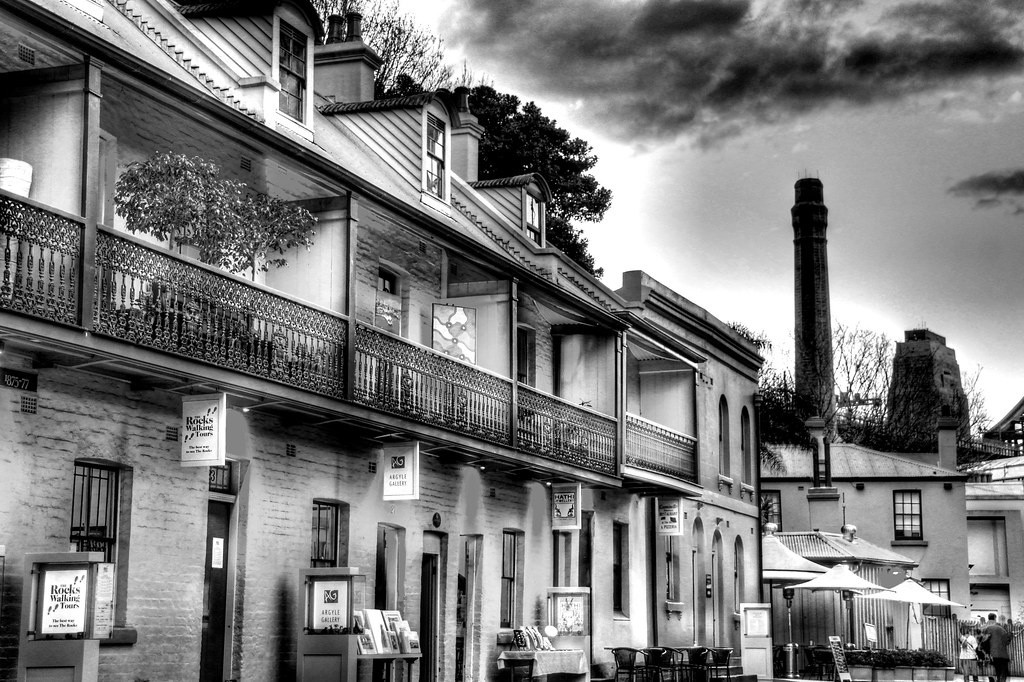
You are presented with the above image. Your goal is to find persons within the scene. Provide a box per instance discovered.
[981,622,1012,682]
[958,625,979,682]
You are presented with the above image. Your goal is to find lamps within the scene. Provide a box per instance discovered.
[969,565,974,569]
[856,483,865,490]
[944,483,952,490]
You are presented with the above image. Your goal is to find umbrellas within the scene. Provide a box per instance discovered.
[855,578,964,650]
[784,564,897,642]
[761,535,832,582]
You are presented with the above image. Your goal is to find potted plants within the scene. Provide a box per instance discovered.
[844,646,956,682]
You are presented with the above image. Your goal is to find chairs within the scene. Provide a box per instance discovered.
[773,643,837,681]
[611,647,734,682]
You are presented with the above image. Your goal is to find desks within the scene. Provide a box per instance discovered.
[497,650,590,682]
[356,652,423,682]
[648,646,702,682]
[816,649,867,682]
